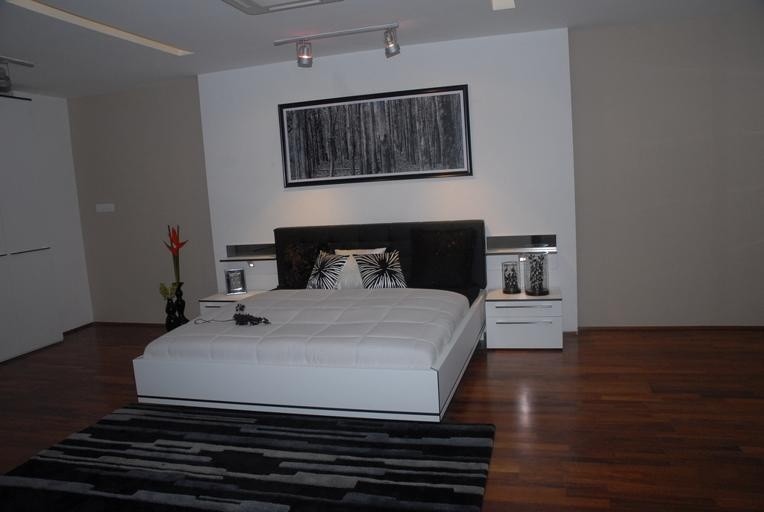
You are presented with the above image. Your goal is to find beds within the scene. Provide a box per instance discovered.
[132,220,488,425]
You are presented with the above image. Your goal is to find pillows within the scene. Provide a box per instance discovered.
[306,248,408,290]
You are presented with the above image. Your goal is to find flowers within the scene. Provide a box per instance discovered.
[162,224,188,284]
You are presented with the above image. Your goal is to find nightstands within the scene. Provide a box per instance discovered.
[197,290,267,318]
[484,289,564,350]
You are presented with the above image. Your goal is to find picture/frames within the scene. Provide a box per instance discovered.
[224,268,247,295]
[277,83,473,188]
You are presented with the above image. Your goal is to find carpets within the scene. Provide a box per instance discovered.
[1,400,498,511]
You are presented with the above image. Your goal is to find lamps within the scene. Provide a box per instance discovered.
[382,25,399,49]
[295,36,313,59]
